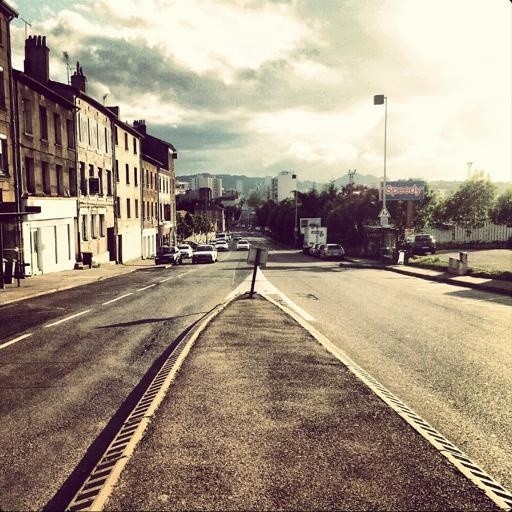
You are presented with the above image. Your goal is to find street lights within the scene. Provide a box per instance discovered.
[374,95,387,208]
[293,175,299,249]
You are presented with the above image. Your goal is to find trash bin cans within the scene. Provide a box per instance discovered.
[83,253,93,268]
[397,250,405,265]
[15,262,25,287]
[459,252,468,275]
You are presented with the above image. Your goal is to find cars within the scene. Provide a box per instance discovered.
[155,233,231,262]
[310,244,345,260]
[413,234,436,255]
[234,234,251,250]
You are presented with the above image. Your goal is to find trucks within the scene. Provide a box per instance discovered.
[303,226,328,253]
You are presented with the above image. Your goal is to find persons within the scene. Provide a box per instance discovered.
[396,240,418,264]
